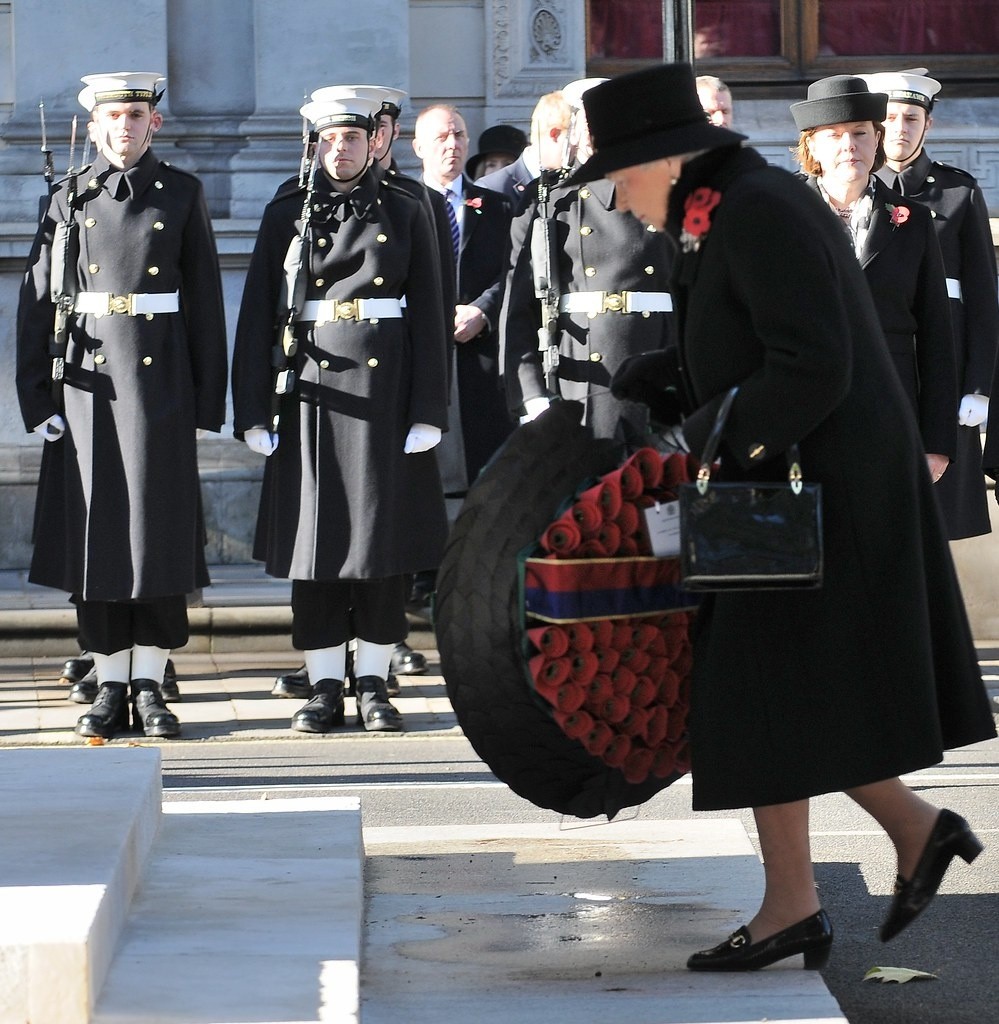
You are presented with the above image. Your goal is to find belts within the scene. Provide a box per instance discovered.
[944,277,962,299]
[296,297,403,322]
[71,290,179,315]
[559,291,672,314]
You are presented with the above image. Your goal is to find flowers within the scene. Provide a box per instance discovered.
[527,449,721,784]
[458,198,483,214]
[884,202,910,231]
[678,187,721,252]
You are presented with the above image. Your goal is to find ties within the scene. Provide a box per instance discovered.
[444,189,459,265]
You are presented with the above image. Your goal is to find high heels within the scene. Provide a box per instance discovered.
[878,807,986,944]
[687,908,834,971]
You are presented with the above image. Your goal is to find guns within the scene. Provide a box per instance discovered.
[269,89,325,432]
[532,115,563,397]
[37,98,93,411]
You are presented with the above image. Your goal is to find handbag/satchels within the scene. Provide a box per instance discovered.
[676,387,824,592]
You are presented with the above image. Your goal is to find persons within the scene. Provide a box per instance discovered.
[15,75,228,737]
[231,67,998,732]
[555,62,998,971]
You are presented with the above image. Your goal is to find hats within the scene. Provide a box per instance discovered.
[77,72,166,113]
[560,77,610,108]
[790,75,889,132]
[298,83,407,132]
[857,66,941,114]
[561,61,747,189]
[463,125,528,180]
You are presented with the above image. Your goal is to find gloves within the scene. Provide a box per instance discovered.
[33,415,66,442]
[243,425,279,456]
[609,348,688,429]
[957,391,991,427]
[403,423,443,454]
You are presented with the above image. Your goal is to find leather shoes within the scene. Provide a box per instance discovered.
[59,637,436,739]
